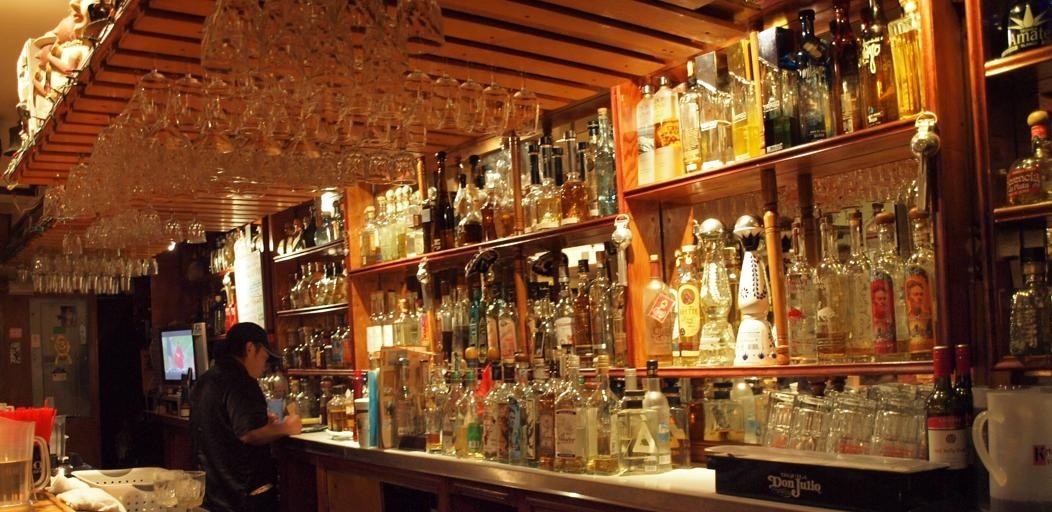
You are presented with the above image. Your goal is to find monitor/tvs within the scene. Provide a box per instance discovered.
[159,327,197,383]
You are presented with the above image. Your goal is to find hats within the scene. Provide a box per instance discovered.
[226,322,282,363]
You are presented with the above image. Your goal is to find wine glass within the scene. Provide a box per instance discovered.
[91,129,413,195]
[118,73,542,148]
[196,1,437,76]
[19,166,208,298]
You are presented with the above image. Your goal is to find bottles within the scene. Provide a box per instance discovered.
[1006,263,1050,357]
[372,199,975,475]
[210,203,344,271]
[1004,108,1050,202]
[360,107,613,264]
[259,257,354,430]
[634,0,925,184]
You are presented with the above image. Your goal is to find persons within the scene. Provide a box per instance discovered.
[906,280,932,337]
[188,322,303,511]
[872,287,895,341]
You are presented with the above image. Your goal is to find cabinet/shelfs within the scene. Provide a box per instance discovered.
[615,0,956,377]
[266,196,352,371]
[963,1,1050,365]
[178,216,266,363]
[348,94,621,371]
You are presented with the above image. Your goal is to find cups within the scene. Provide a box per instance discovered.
[356,398,372,449]
[154,471,207,511]
[971,388,1047,506]
[0,415,51,510]
